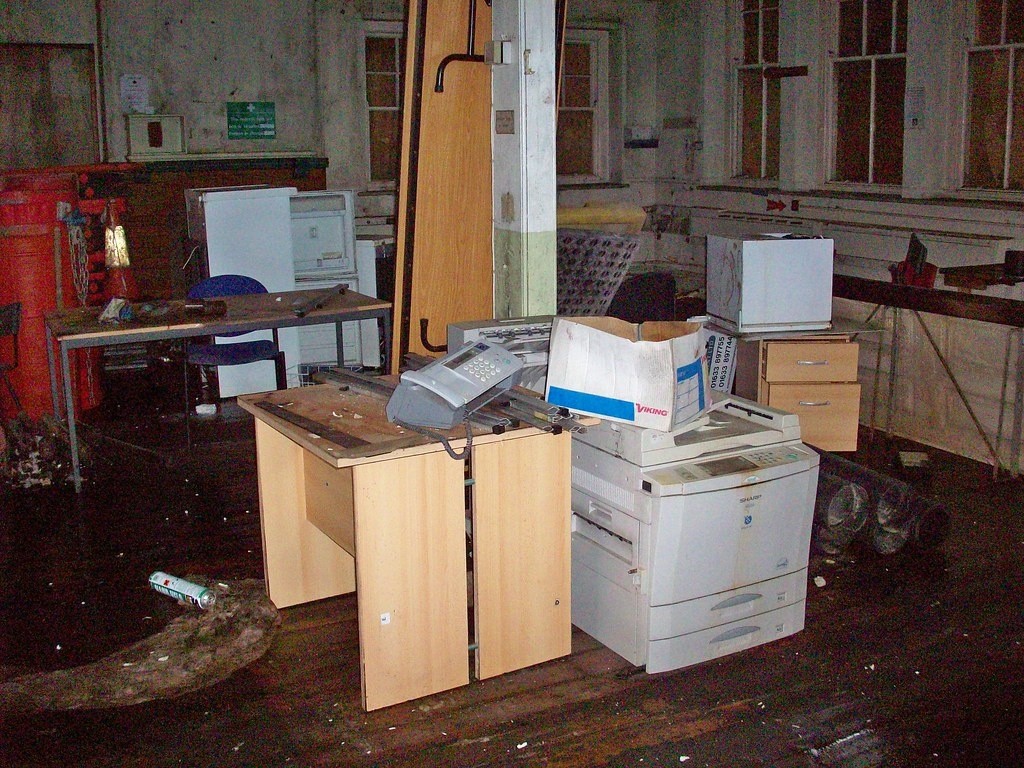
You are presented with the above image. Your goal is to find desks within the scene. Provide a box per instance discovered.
[235,370,604,711]
[42,286,396,494]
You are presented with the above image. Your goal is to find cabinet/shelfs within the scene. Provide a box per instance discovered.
[289,190,365,368]
[706,318,865,456]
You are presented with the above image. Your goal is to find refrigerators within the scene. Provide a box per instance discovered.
[184,180,361,400]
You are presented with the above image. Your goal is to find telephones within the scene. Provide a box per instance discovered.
[385,340,524,432]
